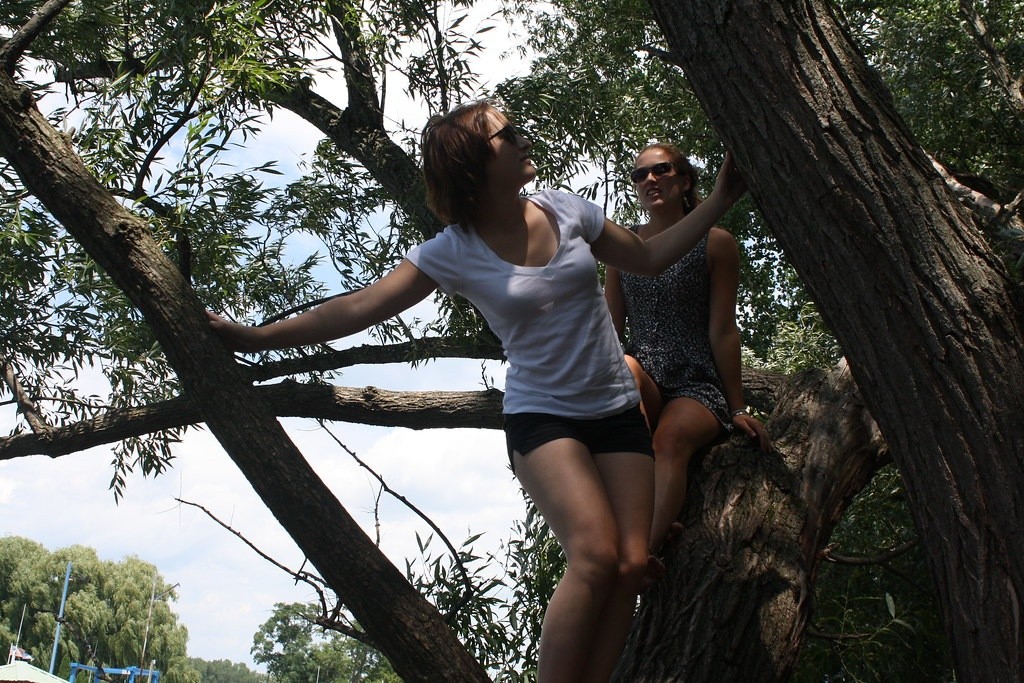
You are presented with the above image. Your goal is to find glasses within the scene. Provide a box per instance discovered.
[489,124,516,145]
[629,161,676,185]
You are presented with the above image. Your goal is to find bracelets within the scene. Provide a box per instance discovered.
[730,408,748,415]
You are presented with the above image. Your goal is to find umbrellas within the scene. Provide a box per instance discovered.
[0,661,71,683]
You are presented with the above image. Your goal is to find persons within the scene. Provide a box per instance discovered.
[604,145,769,594]
[205,99,750,682]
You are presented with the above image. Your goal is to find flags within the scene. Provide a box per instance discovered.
[10,645,32,660]
